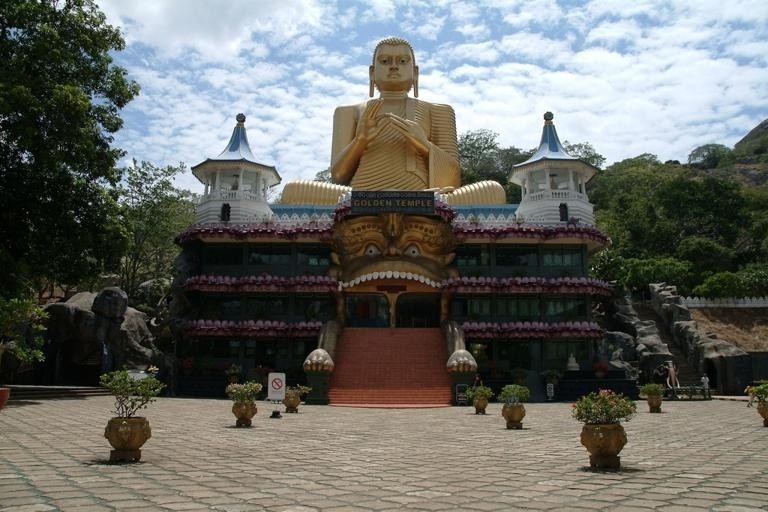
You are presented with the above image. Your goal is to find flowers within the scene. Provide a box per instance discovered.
[571,388,638,424]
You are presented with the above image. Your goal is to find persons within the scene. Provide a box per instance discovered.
[276,36,510,206]
[700,373,711,399]
[744,380,757,407]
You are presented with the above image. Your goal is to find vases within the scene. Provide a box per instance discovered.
[579,424,628,467]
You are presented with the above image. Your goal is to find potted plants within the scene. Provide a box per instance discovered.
[225,380,264,427]
[282,385,312,412]
[99,369,171,463]
[744,380,768,426]
[466,385,495,414]
[497,384,530,429]
[638,383,665,413]
[0,297,51,410]
